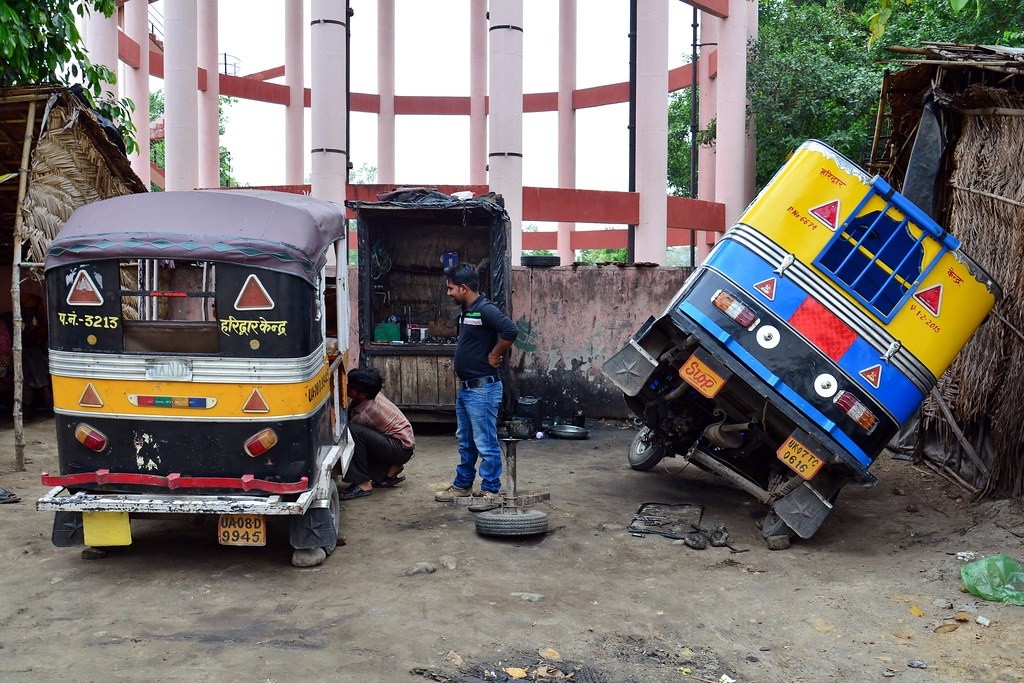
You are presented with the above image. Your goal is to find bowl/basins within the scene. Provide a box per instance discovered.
[547,424,589,438]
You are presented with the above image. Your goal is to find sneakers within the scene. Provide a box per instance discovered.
[468,491,503,511]
[435,485,473,501]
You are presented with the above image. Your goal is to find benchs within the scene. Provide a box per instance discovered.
[123,319,217,353]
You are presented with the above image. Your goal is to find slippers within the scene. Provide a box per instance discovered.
[339,483,373,499]
[372,475,406,487]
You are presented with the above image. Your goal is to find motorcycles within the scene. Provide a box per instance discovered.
[35,193,357,563]
[600,139,1002,545]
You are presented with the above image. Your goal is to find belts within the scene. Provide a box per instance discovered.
[460,375,501,389]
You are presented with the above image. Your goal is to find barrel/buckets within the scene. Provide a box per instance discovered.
[514,396,542,427]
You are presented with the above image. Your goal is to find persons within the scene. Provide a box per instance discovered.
[435,261,519,502]
[337,368,416,499]
[0,487,21,504]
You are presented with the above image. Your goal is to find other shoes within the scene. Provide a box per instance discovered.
[0,487,21,504]
